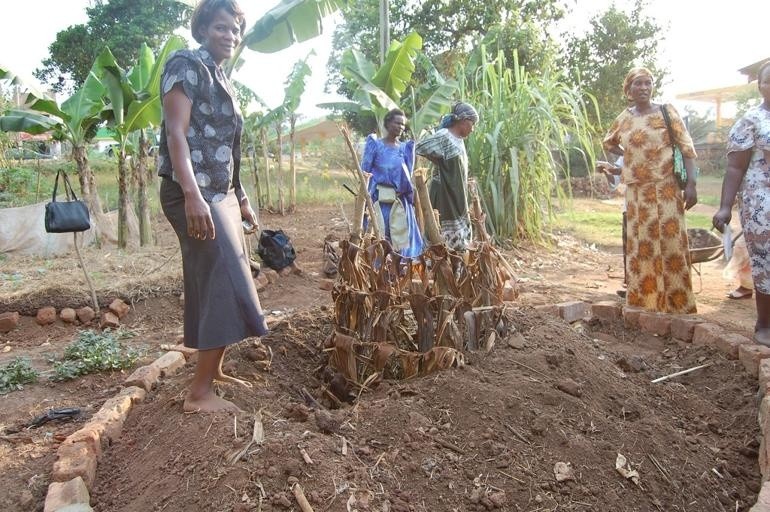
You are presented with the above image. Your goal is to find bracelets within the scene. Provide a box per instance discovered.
[239,194,248,204]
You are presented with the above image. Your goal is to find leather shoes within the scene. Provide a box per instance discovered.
[755,322,770,348]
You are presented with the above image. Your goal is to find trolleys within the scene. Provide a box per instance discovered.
[685,215,749,299]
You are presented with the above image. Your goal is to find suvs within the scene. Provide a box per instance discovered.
[4,147,58,161]
[104,140,137,161]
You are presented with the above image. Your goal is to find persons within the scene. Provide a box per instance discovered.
[593,154,629,289]
[600,63,700,314]
[155,0,272,416]
[354,107,433,280]
[413,101,482,279]
[727,226,755,301]
[709,57,769,349]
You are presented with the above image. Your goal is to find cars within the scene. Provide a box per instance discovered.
[143,144,161,165]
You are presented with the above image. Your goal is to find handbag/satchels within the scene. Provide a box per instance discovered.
[660,104,699,190]
[45,170,90,233]
[322,234,341,275]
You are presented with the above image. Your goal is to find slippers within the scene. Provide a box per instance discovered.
[729,289,752,299]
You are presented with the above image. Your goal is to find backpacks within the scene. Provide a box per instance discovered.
[255,229,296,272]
